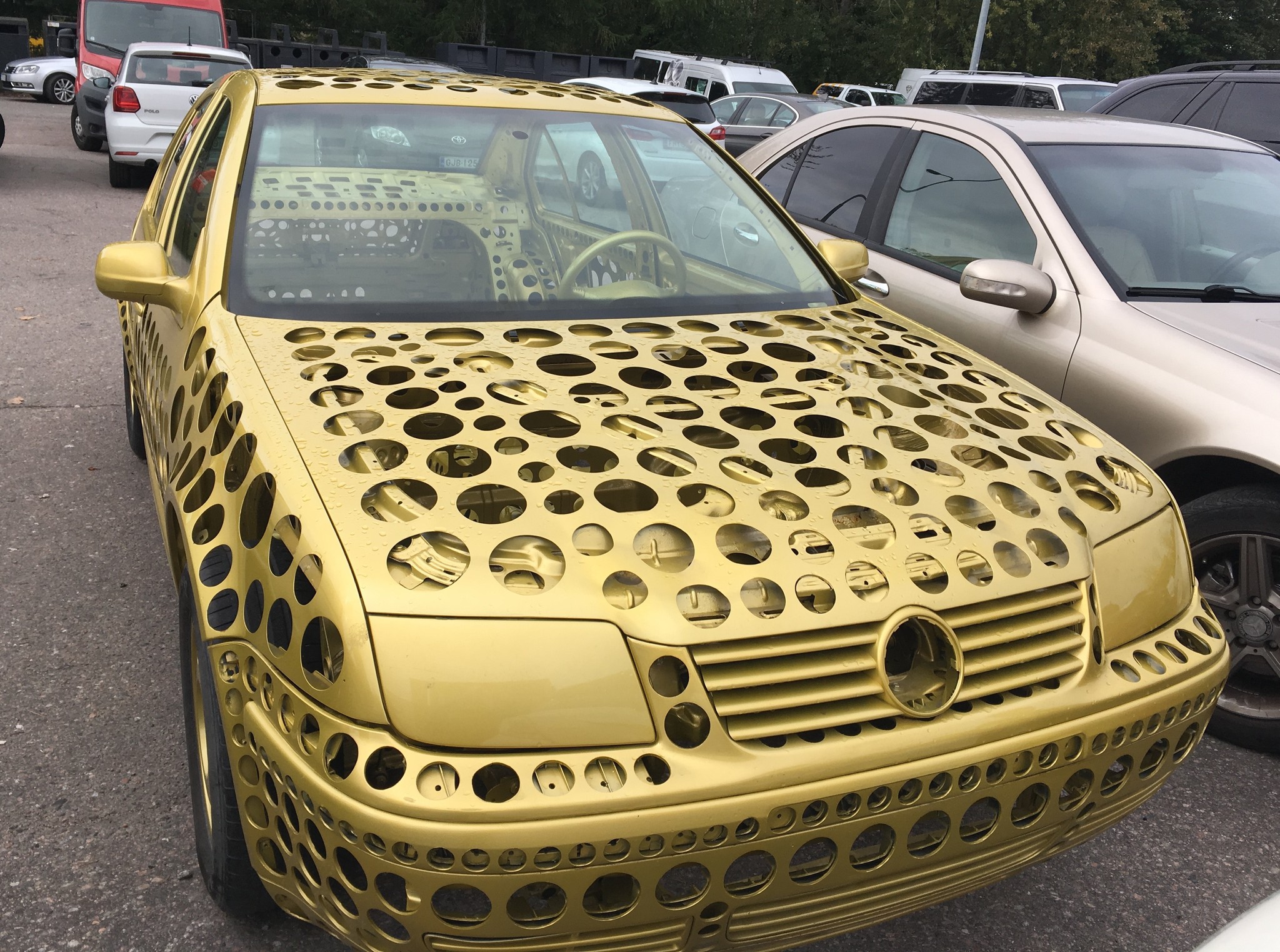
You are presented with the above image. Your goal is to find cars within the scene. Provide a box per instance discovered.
[92,62,1228,952]
[2,54,78,102]
[105,42,250,194]
[709,91,853,157]
[522,76,727,211]
[821,61,1280,159]
[722,104,1280,756]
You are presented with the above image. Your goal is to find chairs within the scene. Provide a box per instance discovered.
[179,70,202,82]
[743,103,766,125]
[1069,176,1157,283]
[208,63,230,80]
[139,63,171,84]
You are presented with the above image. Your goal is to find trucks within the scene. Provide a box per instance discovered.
[69,2,230,145]
[624,47,804,100]
